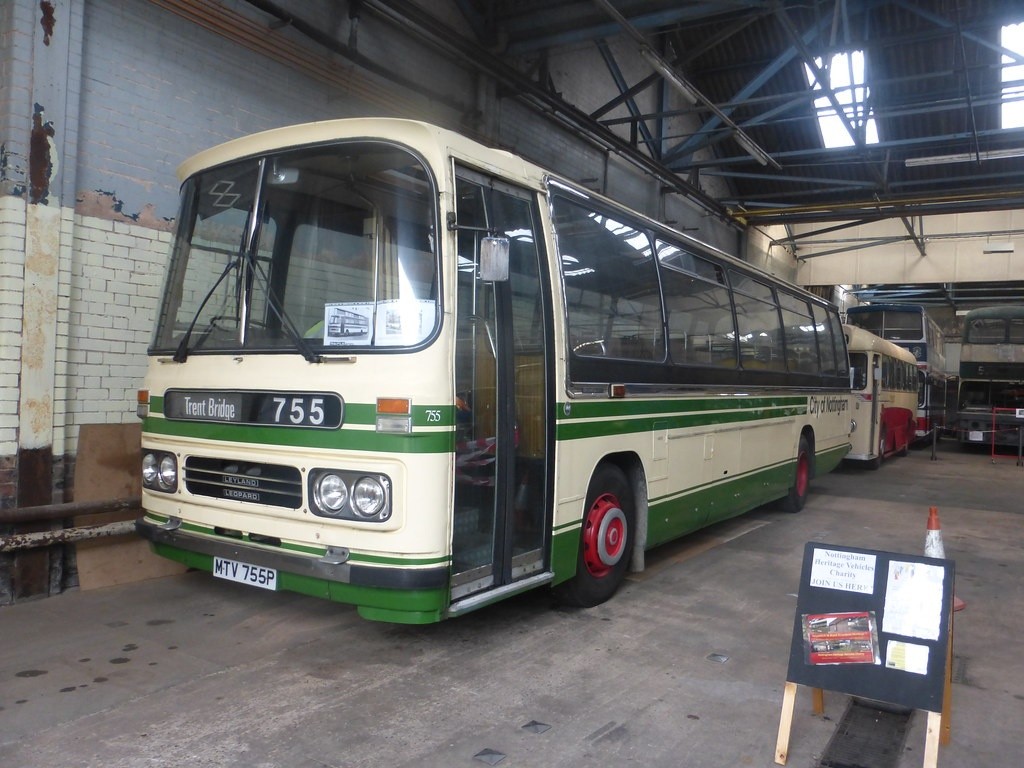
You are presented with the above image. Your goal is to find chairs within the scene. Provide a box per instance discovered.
[471,319,814,455]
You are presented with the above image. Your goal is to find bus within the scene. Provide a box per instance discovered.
[841,324,920,470]
[844,303,946,444]
[328,315,368,337]
[956,306,1024,446]
[137,116,855,626]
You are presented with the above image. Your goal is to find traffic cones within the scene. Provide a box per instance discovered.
[923,507,966,612]
